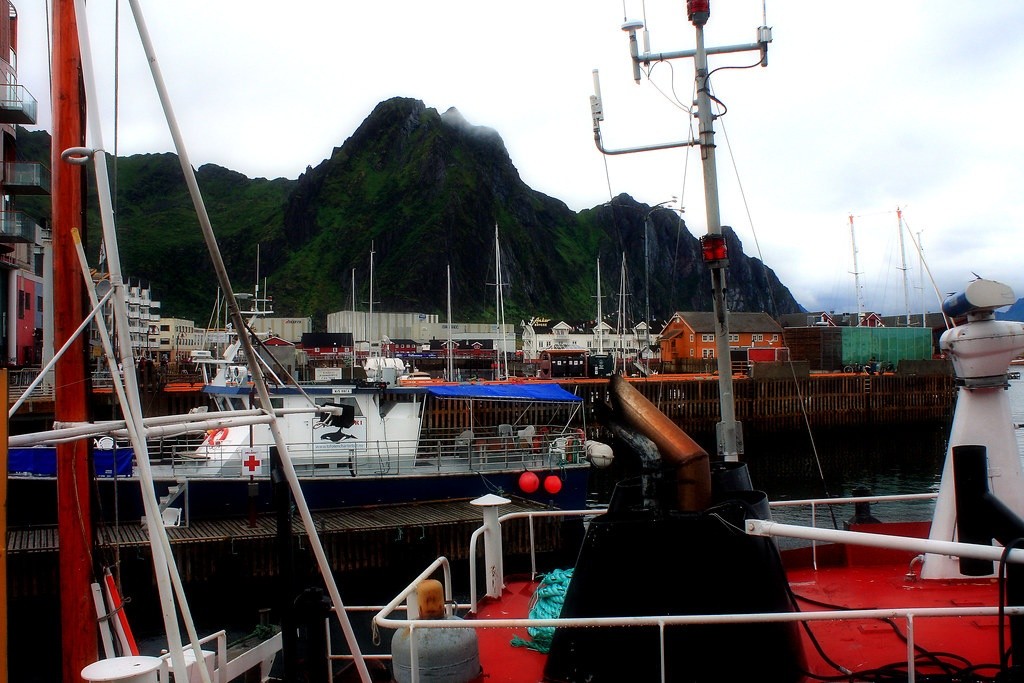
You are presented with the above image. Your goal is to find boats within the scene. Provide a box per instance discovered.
[7,240,614,526]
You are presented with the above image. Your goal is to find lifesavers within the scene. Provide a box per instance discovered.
[208,427,228,445]
[573,427,584,448]
[536,427,548,443]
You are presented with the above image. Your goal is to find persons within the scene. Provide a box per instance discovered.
[8,347,201,387]
